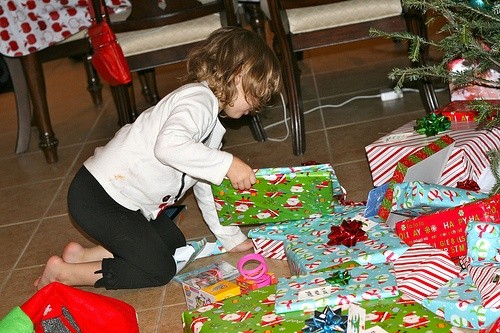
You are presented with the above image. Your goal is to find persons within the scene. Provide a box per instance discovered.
[32,27,283,292]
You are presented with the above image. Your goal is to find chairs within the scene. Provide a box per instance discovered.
[0,0,439,163]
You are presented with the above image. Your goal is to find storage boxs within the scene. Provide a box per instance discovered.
[182,58,500,333]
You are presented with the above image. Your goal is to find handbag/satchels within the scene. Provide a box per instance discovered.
[88,0,133,86]
